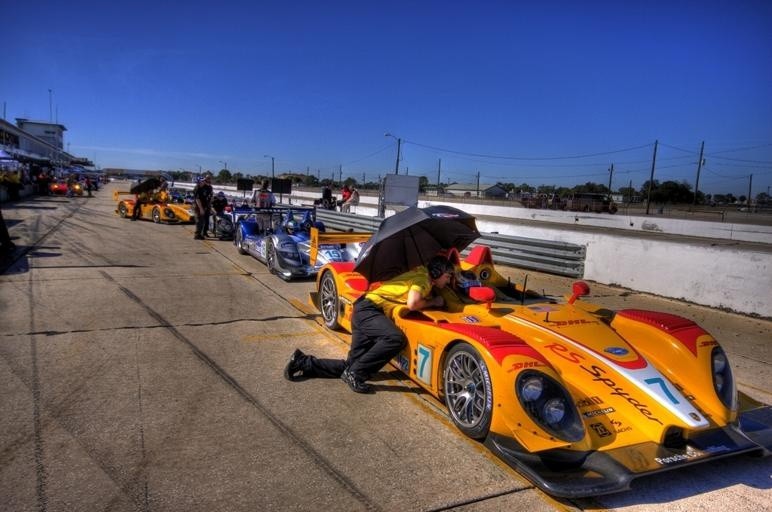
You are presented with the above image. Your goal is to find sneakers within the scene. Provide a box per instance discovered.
[340,365,371,394]
[282,348,306,381]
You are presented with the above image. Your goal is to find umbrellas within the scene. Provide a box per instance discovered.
[351,205,482,284]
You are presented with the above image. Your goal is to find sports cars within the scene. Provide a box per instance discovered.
[235,203,357,283]
[112,183,255,240]
[48,176,84,199]
[308,224,771,500]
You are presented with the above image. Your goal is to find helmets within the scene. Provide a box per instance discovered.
[453,270,482,306]
[286,220,300,233]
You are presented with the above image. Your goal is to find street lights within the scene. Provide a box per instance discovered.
[383,131,401,174]
[218,160,227,186]
[264,155,275,179]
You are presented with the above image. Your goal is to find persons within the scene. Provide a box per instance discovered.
[131,173,228,240]
[250,178,276,234]
[64,173,76,197]
[283,255,456,393]
[337,184,351,212]
[342,184,359,213]
[84,174,92,197]
